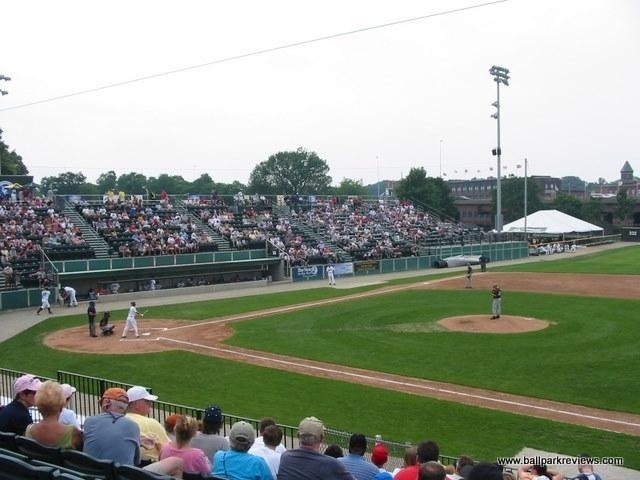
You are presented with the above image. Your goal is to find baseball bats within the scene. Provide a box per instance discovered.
[141,309,149,316]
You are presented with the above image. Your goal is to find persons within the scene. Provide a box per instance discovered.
[122,302,144,338]
[87,288,99,302]
[59,286,78,307]
[88,302,98,337]
[489,284,503,320]
[0,372,602,480]
[1,189,577,294]
[99,310,115,336]
[37,287,52,315]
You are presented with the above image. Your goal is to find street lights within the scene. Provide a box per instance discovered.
[488,66,510,233]
[439,139,444,179]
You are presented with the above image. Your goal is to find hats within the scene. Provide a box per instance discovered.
[14,373,387,464]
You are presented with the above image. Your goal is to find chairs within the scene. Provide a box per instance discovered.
[0,430,224,479]
